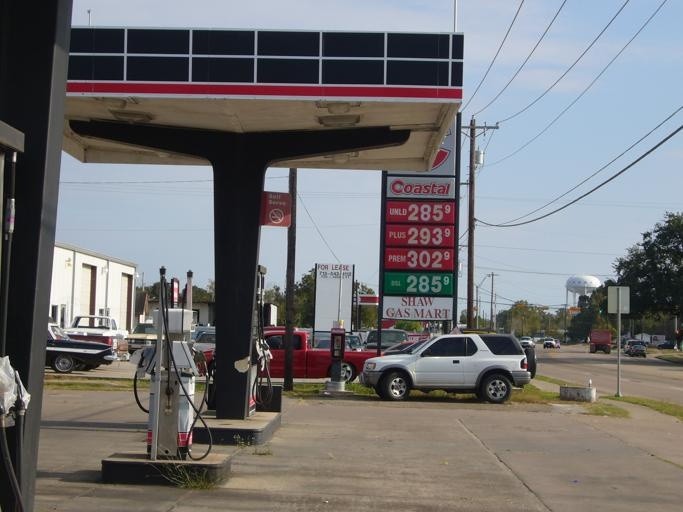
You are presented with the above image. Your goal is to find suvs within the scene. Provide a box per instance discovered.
[360,331,538,405]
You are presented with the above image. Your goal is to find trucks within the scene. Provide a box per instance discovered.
[589,328,612,354]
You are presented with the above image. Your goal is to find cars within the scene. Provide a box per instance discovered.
[519,336,561,350]
[616,335,646,358]
[656,341,674,349]
